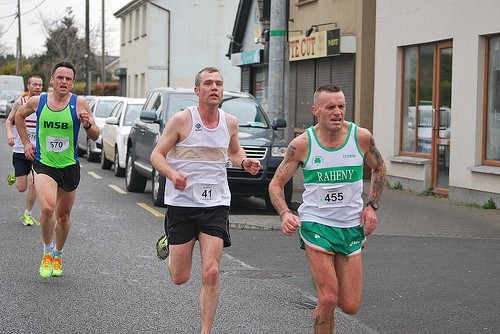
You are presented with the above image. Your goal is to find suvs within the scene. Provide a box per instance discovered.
[124,86,294,213]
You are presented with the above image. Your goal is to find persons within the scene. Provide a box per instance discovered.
[15,60,100,279]
[4,73,43,226]
[156,65,261,334]
[268,84,386,334]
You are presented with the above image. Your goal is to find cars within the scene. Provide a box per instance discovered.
[408,105,451,164]
[0,90,22,117]
[5,92,25,119]
[100,96,148,178]
[75,94,127,163]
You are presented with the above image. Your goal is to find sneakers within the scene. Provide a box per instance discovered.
[39,251,54,279]
[6,172,16,185]
[156,234,169,260]
[51,256,63,277]
[19,214,40,226]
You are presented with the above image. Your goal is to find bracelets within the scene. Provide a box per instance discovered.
[279,211,292,221]
[241,159,245,170]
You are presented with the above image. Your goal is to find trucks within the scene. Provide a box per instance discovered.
[0,74,25,103]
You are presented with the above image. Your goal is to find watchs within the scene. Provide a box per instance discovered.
[366,200,379,210]
[82,122,92,129]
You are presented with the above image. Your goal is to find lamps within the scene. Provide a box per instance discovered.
[266,30,302,42]
[305,22,337,37]
[254,38,266,46]
